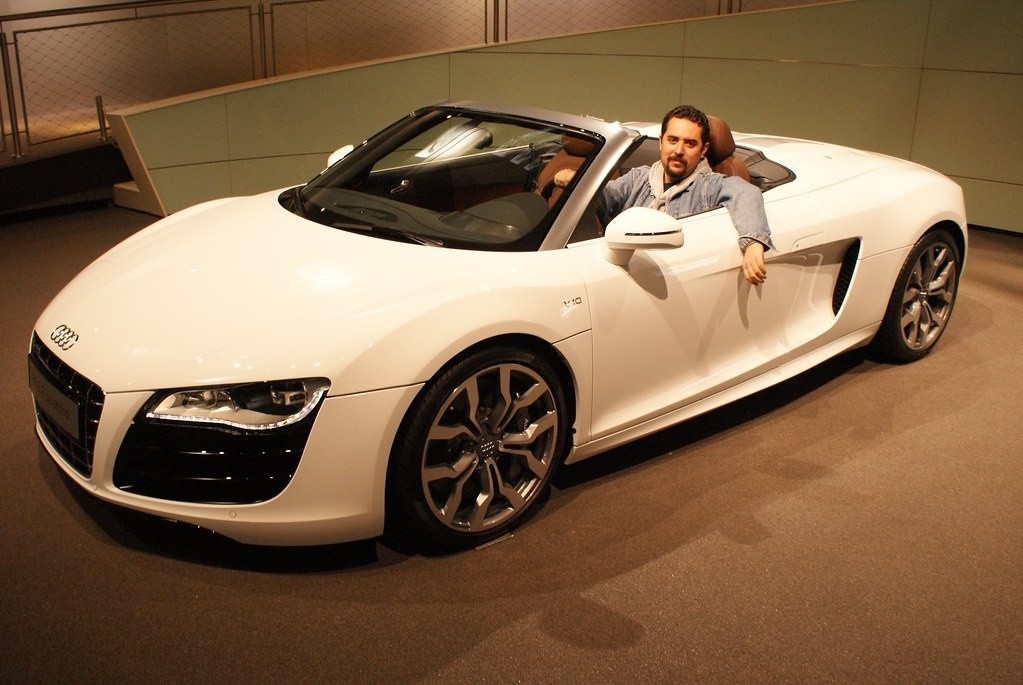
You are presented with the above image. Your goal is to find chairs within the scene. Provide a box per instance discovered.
[534,135,620,237]
[704,114,750,184]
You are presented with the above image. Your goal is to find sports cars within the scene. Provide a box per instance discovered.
[26,96,971,558]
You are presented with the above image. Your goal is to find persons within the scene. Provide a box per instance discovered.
[555,104,776,286]
